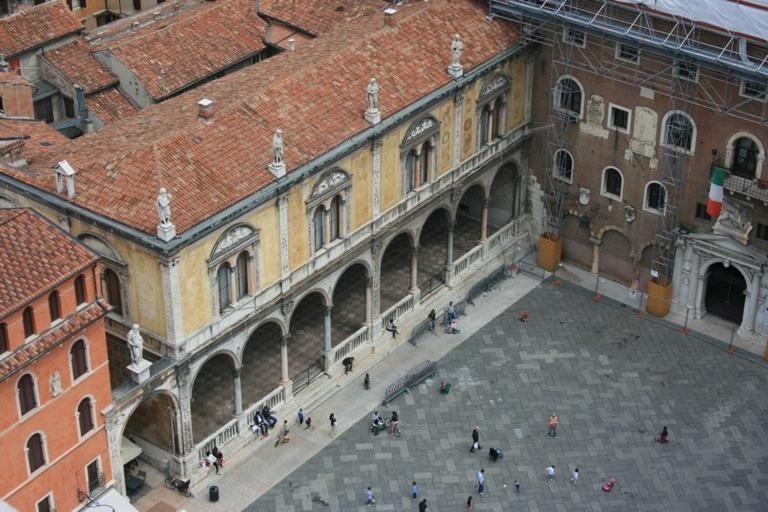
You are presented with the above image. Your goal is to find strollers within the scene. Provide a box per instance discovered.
[488,448,504,462]
[275,432,290,447]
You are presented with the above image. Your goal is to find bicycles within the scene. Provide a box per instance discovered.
[164,471,192,498]
[385,418,401,437]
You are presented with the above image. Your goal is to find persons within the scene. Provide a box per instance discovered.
[656,426,670,444]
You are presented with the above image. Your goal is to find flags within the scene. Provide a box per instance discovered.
[706,166,730,218]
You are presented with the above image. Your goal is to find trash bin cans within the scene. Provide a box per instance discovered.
[209,486,218,502]
